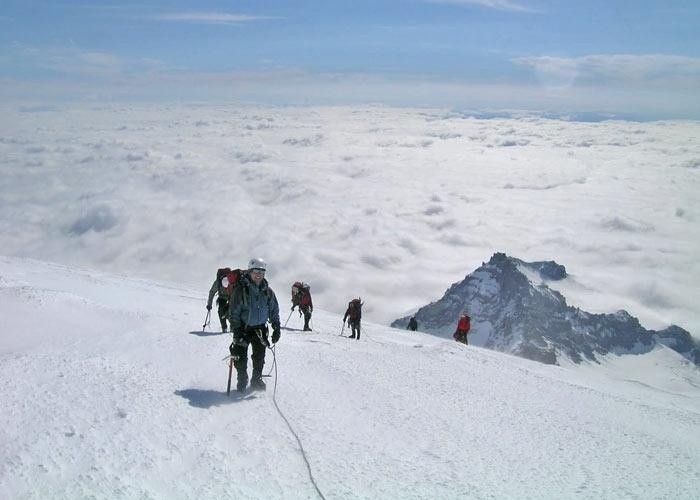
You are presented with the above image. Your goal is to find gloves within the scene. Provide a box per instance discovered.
[207,304,213,311]
[271,323,281,343]
[233,328,248,347]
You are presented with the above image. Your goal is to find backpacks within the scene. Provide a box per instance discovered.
[216,267,230,295]
[228,269,269,311]
[292,282,303,306]
[352,298,361,320]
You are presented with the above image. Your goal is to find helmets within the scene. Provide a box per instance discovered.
[248,257,266,270]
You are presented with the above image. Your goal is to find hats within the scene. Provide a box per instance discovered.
[222,277,229,287]
[302,282,310,289]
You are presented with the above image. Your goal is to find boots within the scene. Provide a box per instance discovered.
[237,368,266,392]
[304,324,313,331]
[221,319,228,332]
[349,329,361,340]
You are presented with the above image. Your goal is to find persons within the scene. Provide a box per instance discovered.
[406,317,418,331]
[343,302,362,340]
[227,258,281,392]
[291,283,314,331]
[456,310,471,345]
[206,268,235,333]
